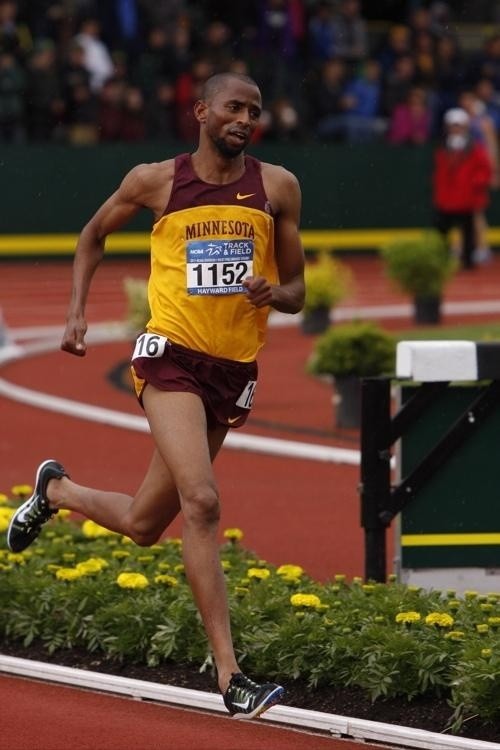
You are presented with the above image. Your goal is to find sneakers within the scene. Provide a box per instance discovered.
[8,460,69,554]
[223,673,284,721]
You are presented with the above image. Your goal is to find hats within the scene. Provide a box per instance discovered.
[445,109,470,128]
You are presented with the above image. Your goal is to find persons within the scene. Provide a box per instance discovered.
[7,72,305,721]
[0,1,500,269]
